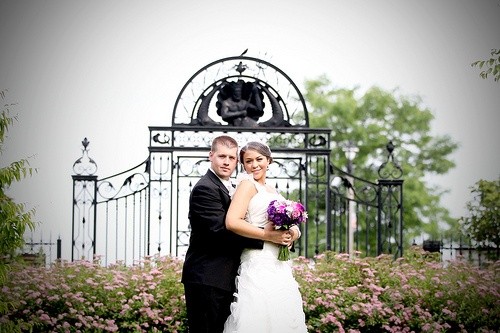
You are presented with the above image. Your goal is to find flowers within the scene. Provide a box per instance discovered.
[266,199,308,260]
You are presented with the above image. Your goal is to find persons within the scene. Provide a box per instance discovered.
[180,136,300,333]
[225,141,311,333]
